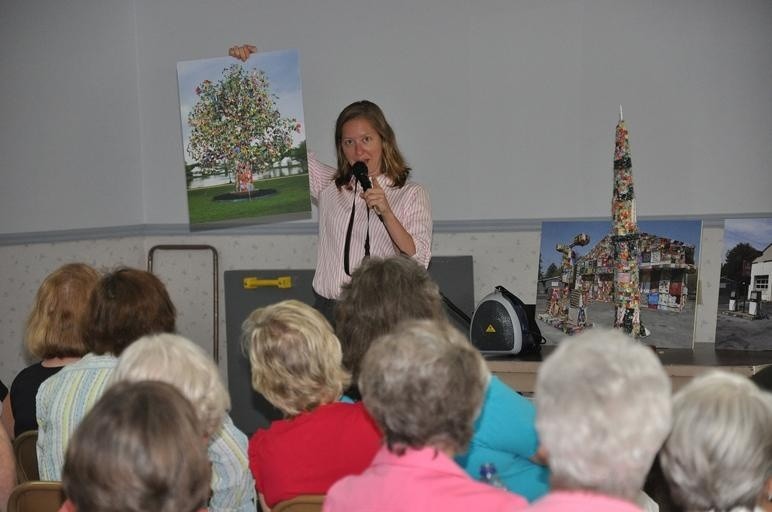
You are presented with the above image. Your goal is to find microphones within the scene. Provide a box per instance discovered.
[353,159,384,223]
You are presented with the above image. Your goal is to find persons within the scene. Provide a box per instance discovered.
[227,39,433,335]
[0,251,770,512]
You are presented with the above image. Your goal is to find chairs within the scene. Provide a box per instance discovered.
[272,493,327,512]
[6,428,64,512]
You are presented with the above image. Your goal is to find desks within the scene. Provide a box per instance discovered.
[484,344,771,393]
